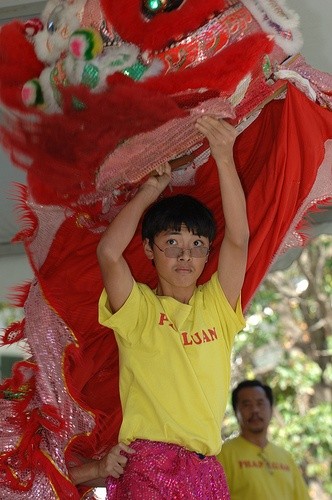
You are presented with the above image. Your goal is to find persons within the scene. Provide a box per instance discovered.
[216,379,312,500]
[99,115,251,500]
[70,442,136,486]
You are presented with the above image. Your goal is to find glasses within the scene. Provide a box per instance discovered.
[152,241,211,259]
